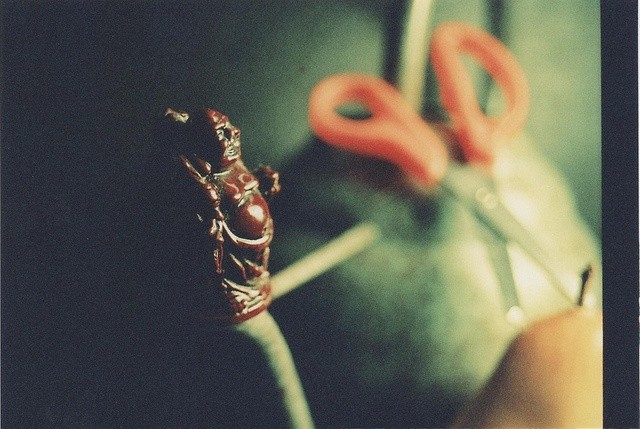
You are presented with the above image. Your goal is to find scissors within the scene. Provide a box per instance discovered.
[308,21,594,329]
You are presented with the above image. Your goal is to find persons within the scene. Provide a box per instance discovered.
[171,108,281,325]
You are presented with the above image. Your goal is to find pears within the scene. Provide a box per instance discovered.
[449,266,602,429]
